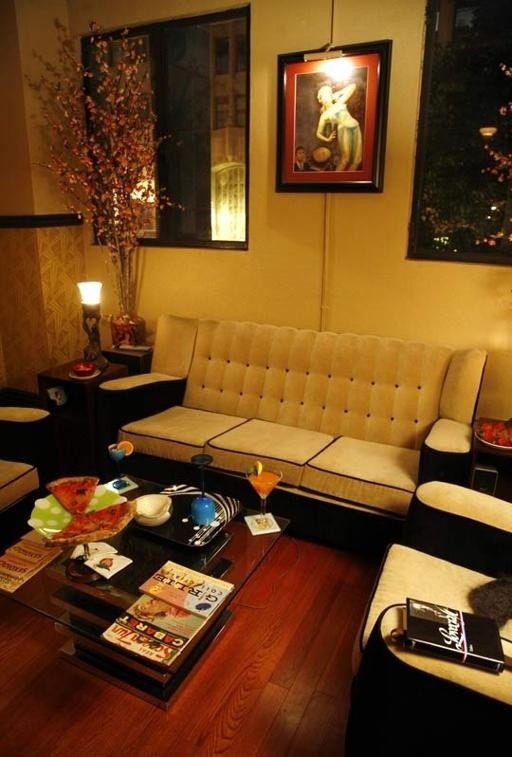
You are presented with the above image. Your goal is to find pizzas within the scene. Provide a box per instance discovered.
[45,476,137,546]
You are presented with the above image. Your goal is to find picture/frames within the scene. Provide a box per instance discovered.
[275,39,393,195]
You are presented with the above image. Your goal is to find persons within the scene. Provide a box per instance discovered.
[315,80,361,172]
[295,146,315,171]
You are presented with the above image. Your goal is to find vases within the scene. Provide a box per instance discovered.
[109,316,147,347]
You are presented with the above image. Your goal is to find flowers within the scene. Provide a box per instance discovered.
[24,17,187,321]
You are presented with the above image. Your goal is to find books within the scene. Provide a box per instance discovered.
[101,560,234,667]
[0,530,65,594]
[402,597,505,674]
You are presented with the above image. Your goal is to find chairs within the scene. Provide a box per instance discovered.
[1,406,49,519]
[339,479,512,756]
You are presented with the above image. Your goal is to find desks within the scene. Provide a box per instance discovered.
[37,359,129,467]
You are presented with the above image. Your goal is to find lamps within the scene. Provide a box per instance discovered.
[76,281,109,372]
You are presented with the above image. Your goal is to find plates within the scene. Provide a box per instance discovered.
[473,428,512,451]
[68,370,102,380]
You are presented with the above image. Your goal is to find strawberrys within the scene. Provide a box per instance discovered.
[100,558,113,568]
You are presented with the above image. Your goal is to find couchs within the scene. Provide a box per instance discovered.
[99,314,489,559]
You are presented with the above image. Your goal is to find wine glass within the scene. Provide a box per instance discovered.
[109,442,130,490]
[246,464,284,530]
[189,454,216,526]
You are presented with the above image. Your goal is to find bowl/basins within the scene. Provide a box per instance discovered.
[130,493,175,528]
[70,361,97,376]
